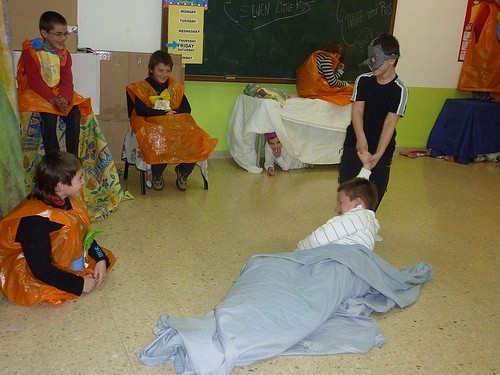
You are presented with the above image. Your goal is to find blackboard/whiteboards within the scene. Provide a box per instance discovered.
[160,0,398,84]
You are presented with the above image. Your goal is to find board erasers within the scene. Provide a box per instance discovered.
[225,75,237,80]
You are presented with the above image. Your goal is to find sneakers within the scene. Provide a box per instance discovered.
[175,165,187,191]
[152,171,164,191]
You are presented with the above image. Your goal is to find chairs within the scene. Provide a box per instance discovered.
[121,88,208,195]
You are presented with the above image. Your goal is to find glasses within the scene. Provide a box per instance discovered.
[49,32,70,38]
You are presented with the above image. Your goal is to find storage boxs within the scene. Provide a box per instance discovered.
[0,0,78,54]
[94,52,186,165]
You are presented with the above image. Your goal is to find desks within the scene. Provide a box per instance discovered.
[226,94,353,174]
[427,98,500,165]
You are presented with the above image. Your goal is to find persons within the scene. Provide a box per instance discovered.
[337,35,409,212]
[264,132,313,176]
[0,151,117,306]
[136,149,380,374]
[121,50,218,191]
[16,11,92,158]
[295,40,354,107]
